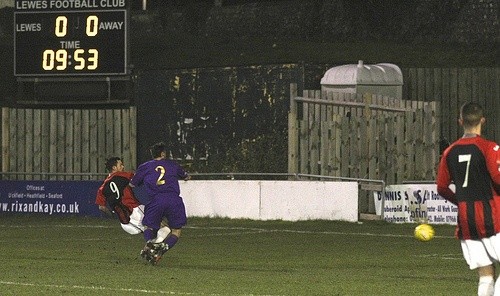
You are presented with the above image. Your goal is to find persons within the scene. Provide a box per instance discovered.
[128,141,191,263]
[95,156,172,253]
[437,102,500,295]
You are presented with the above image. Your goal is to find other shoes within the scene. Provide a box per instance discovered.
[140,242,163,266]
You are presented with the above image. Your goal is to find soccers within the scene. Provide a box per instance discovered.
[414,224,435,242]
